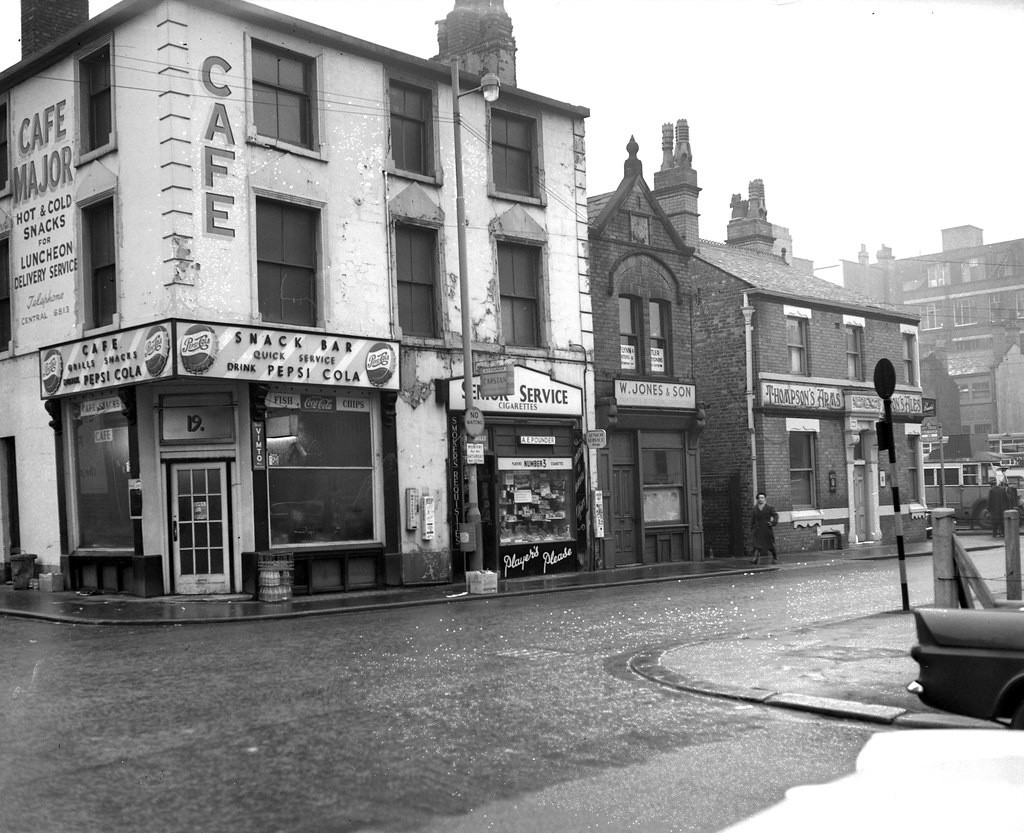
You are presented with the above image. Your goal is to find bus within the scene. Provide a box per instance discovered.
[923,451,1019,528]
[1006,474,1024,525]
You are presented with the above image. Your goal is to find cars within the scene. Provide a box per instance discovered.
[718,730,1024,832]
[906,606,1024,731]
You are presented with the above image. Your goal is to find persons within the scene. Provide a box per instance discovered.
[987,481,1015,537]
[748,492,779,563]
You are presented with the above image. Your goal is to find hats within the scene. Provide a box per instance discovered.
[756,493,766,500]
[991,481,996,485]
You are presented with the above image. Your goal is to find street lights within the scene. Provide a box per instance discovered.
[446,52,503,594]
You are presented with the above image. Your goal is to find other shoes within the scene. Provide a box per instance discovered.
[772,556,777,562]
[750,557,758,564]
